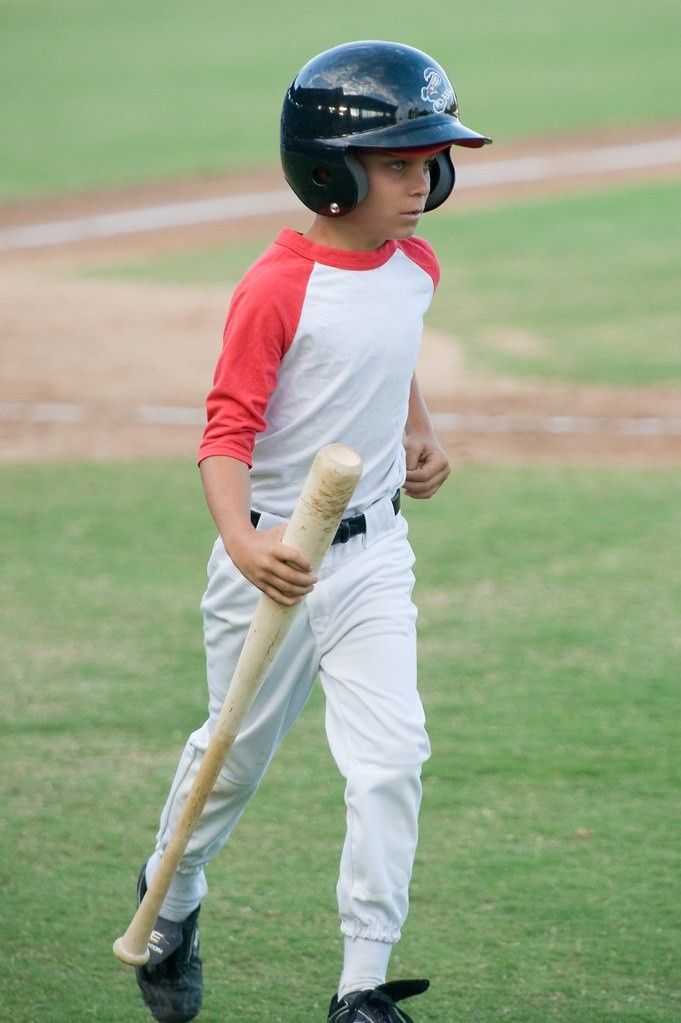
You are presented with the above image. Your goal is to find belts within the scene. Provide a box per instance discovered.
[248,490,405,546]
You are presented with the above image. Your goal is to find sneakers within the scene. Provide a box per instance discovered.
[325,978,429,1023]
[136,864,206,1022]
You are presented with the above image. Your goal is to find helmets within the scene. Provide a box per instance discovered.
[280,40,493,216]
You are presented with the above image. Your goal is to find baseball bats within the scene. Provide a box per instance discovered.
[111,441,365,968]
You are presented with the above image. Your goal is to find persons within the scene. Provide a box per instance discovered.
[134,38,494,1023]
